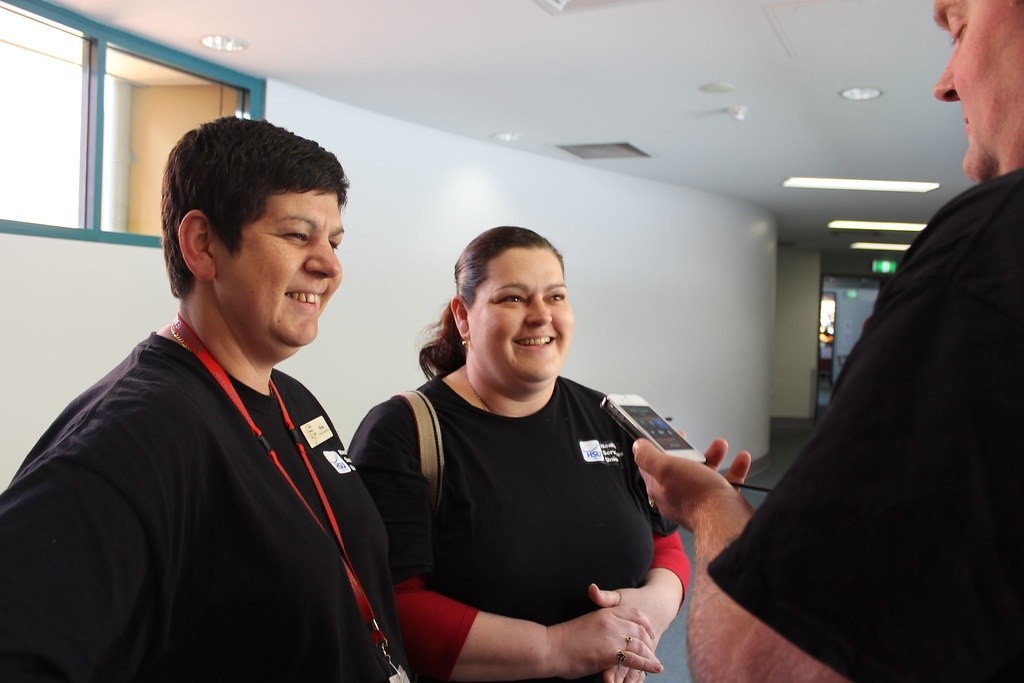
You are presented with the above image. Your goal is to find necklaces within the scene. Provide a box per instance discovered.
[170,324,274,396]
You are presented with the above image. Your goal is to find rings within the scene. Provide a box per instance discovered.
[616,651,626,665]
[648,497,658,512]
[626,637,632,646]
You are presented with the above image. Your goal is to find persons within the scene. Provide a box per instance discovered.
[632,0,1024,683]
[343,226,690,683]
[0,117,411,683]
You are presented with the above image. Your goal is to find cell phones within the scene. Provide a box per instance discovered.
[600,394,707,467]
